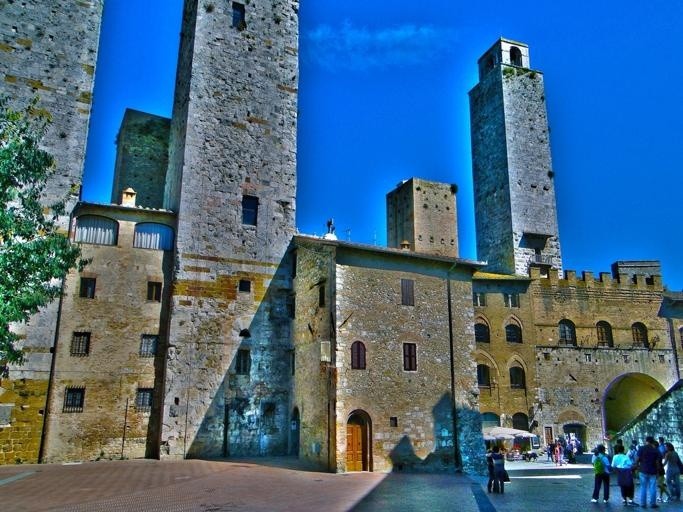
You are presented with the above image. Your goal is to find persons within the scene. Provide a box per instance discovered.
[484,431,683,509]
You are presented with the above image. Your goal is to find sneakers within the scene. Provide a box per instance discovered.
[590,499,598,503]
[628,501,639,507]
[642,504,647,508]
[658,496,680,505]
[604,500,608,504]
[623,501,627,506]
[652,505,658,509]
[488,489,504,495]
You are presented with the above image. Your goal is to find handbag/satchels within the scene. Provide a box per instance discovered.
[502,470,510,483]
[594,456,605,475]
